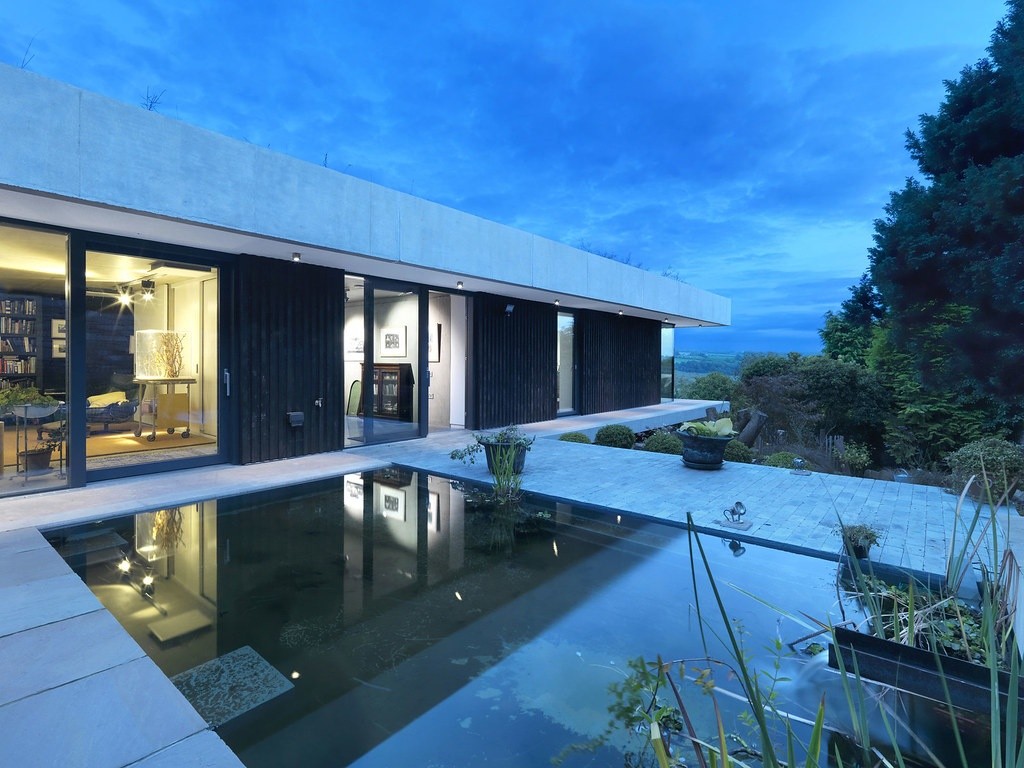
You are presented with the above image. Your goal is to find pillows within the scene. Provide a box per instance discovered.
[87,391,129,408]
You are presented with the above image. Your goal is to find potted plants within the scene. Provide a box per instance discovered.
[842,561,858,580]
[947,438,1024,504]
[448,481,552,524]
[840,524,881,558]
[449,423,535,475]
[676,418,739,470]
[0,384,66,418]
[16,424,67,473]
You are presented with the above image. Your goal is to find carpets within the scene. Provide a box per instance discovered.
[348,430,430,445]
[53,446,205,480]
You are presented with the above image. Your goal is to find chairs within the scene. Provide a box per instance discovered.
[86,371,145,434]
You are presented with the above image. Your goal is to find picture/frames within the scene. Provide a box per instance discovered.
[380,326,407,357]
[379,486,406,521]
[428,323,439,362]
[51,339,66,359]
[427,492,438,533]
[51,318,66,339]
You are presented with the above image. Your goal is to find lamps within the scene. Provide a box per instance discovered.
[793,458,804,471]
[554,300,560,305]
[120,286,133,304]
[142,279,154,300]
[723,502,746,524]
[142,576,154,594]
[665,318,668,322]
[699,325,702,327]
[345,293,350,302]
[457,281,464,288]
[119,560,133,575]
[721,538,746,557]
[619,310,623,315]
[292,253,301,261]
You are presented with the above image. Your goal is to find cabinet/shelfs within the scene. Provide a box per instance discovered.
[0,292,42,396]
[360,464,412,489]
[359,363,413,422]
[134,379,198,442]
